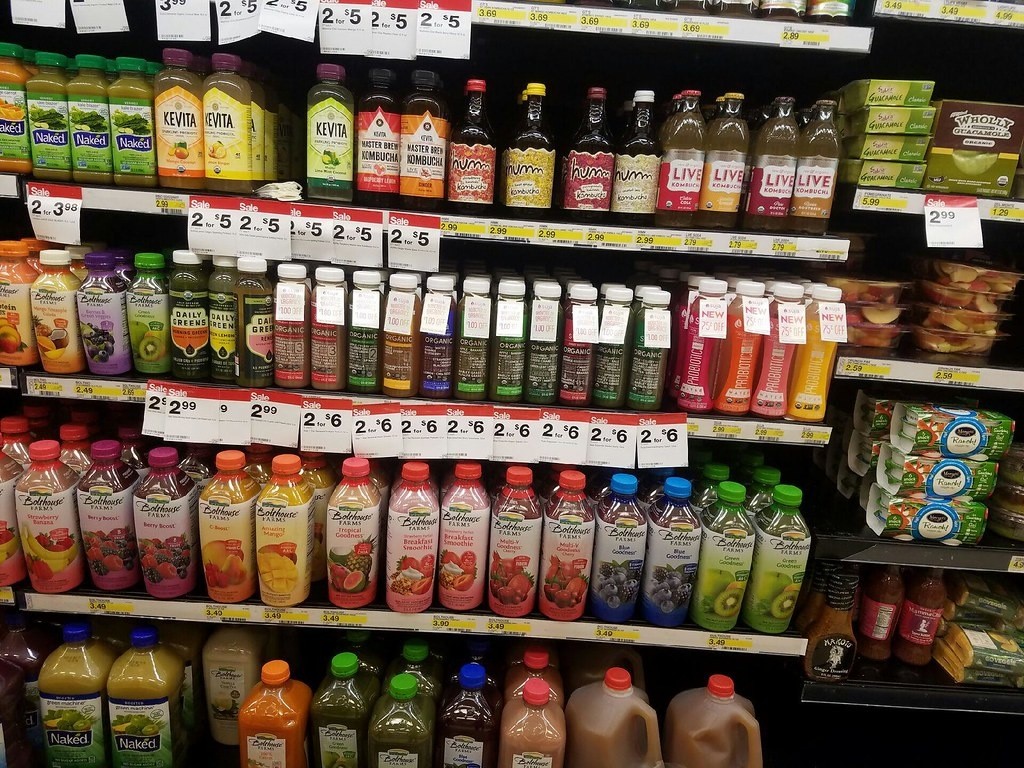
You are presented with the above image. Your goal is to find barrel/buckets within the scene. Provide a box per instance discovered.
[566,635,764,768]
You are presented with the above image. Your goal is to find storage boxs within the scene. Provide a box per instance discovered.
[820,78,1024,691]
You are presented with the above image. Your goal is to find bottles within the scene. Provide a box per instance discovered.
[0,232,275,387]
[277,251,672,410]
[154,48,303,197]
[323,449,806,635]
[562,86,841,232]
[306,64,496,216]
[802,558,948,684]
[0,615,566,768]
[0,42,160,186]
[0,400,338,609]
[500,81,556,214]
[659,268,843,423]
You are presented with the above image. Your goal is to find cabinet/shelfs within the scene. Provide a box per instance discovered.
[0,0,873,665]
[774,0,1024,720]
